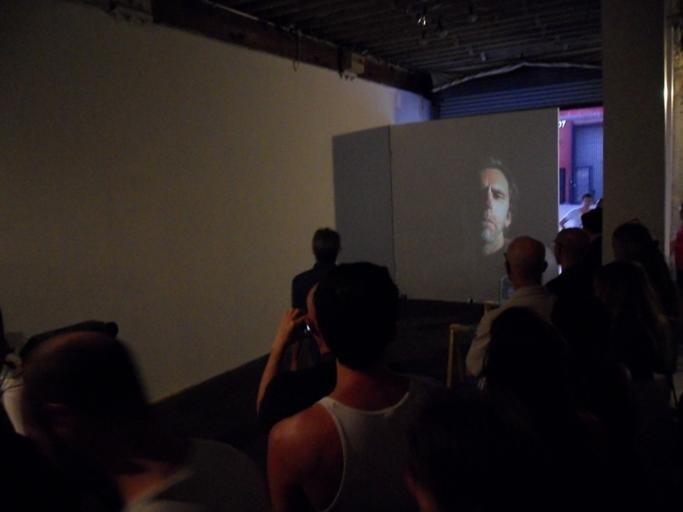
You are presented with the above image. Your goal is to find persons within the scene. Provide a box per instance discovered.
[254,280,338,466]
[0,319,215,512]
[451,218,680,510]
[268,263,464,508]
[401,153,514,302]
[558,192,593,232]
[292,226,342,373]
